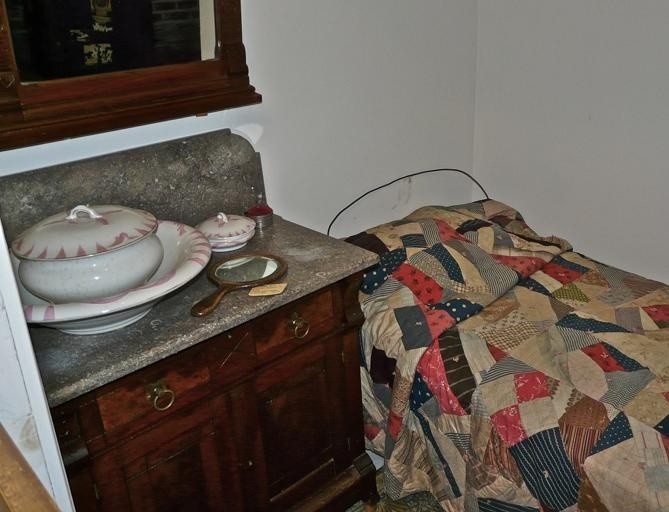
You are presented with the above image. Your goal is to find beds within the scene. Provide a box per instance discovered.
[339,198,669,512]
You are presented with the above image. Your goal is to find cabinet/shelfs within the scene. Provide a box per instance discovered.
[49,270,380,512]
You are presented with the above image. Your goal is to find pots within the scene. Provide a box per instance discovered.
[12,202,165,301]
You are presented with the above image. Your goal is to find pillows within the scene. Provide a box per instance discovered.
[343,199,527,294]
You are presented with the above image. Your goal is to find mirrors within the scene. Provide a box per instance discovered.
[0,0,262,152]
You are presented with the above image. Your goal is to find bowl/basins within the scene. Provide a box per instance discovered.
[196,211,255,251]
[9,219,212,337]
